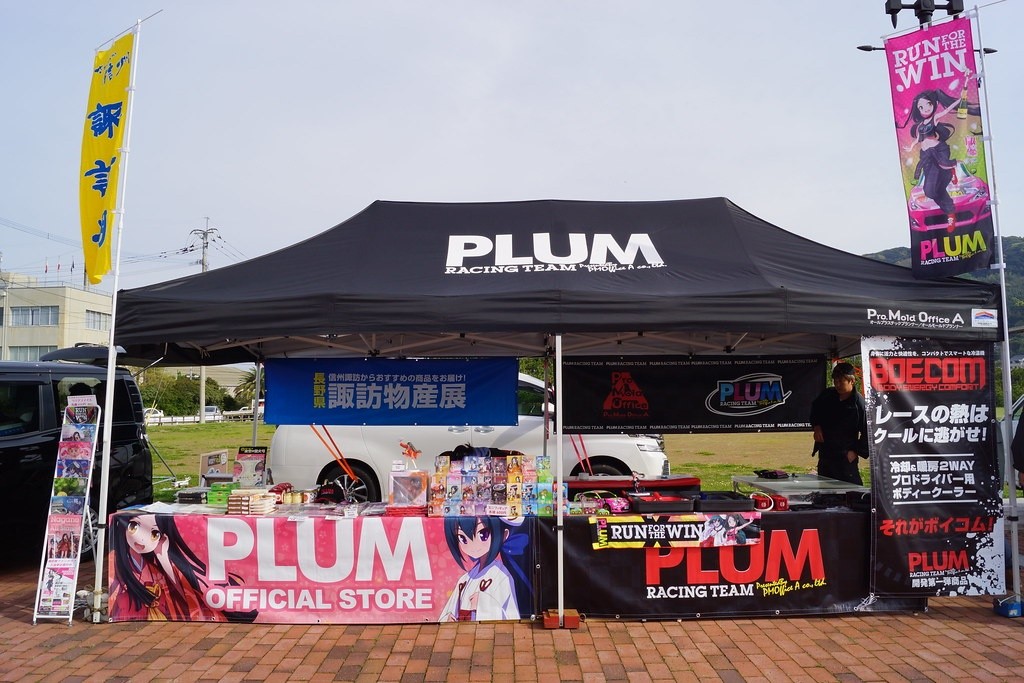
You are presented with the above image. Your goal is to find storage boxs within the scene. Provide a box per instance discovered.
[680,490,754,512]
[206,482,240,503]
[623,491,696,514]
[428,455,569,517]
[388,468,428,507]
[177,487,211,504]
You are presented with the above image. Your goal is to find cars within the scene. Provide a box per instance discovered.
[907,160,992,233]
[999,393,1024,491]
[197,405,221,423]
[144,408,164,418]
[266,370,672,504]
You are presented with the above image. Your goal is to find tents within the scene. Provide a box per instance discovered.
[93,198,1024,632]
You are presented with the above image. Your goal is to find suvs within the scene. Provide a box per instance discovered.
[0,346,154,564]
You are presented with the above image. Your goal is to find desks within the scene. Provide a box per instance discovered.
[532,513,929,619]
[109,501,535,624]
[730,473,871,496]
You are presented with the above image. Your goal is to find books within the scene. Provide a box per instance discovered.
[38,404,98,616]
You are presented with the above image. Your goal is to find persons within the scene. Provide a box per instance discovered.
[809,363,869,488]
[1011,408,1024,496]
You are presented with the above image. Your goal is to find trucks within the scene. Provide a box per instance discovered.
[222,399,265,421]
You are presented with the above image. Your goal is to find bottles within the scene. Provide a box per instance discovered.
[957,86,968,120]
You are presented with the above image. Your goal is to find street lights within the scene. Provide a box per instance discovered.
[856,0,1000,57]
